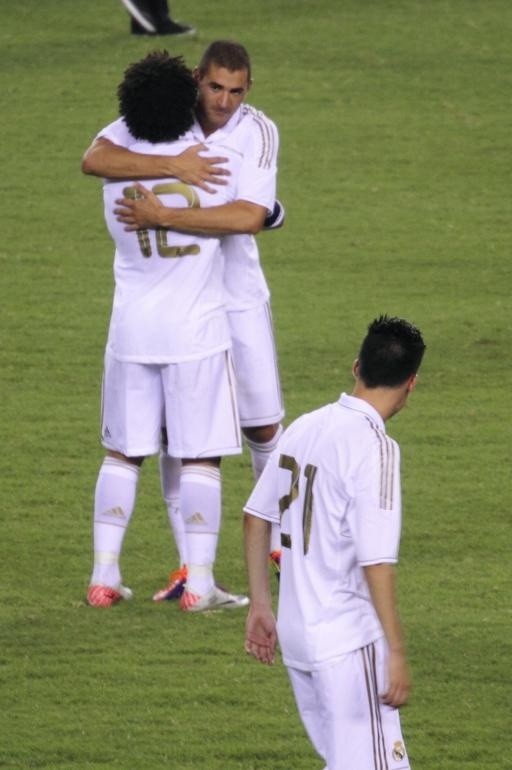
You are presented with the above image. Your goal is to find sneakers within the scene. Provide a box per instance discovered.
[131,19,196,35]
[152,564,250,612]
[86,584,132,608]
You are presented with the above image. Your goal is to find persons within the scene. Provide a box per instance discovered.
[127,1,194,39]
[82,47,253,615]
[242,311,428,769]
[80,36,287,605]
